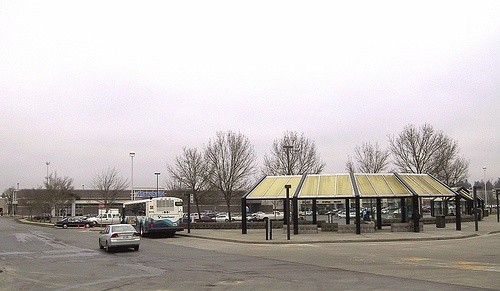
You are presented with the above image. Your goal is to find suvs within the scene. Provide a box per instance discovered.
[87,212,122,227]
[34,213,51,222]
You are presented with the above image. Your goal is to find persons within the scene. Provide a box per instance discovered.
[363,209,367,218]
[364,211,374,224]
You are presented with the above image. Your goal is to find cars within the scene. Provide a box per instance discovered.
[54,216,96,228]
[182,205,401,223]
[98,224,141,254]
[421,204,466,216]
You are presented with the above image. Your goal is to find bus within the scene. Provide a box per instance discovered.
[118,197,184,238]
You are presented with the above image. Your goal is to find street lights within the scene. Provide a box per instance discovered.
[283,146,294,175]
[154,173,160,198]
[129,152,135,201]
[482,166,487,209]
